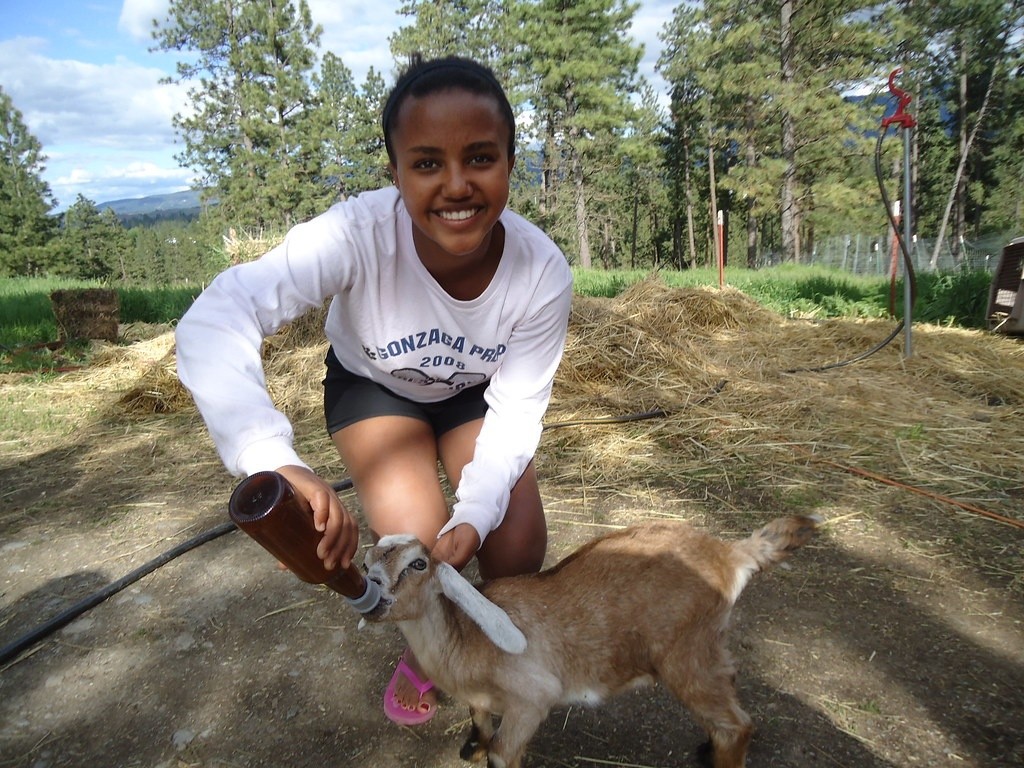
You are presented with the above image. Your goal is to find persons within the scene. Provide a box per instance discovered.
[176,51,573,725]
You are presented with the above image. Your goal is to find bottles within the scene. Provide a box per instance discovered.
[227,469,383,617]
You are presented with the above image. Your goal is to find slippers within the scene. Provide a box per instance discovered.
[383,657,439,725]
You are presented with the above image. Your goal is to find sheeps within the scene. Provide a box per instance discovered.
[355,513,827,768]
